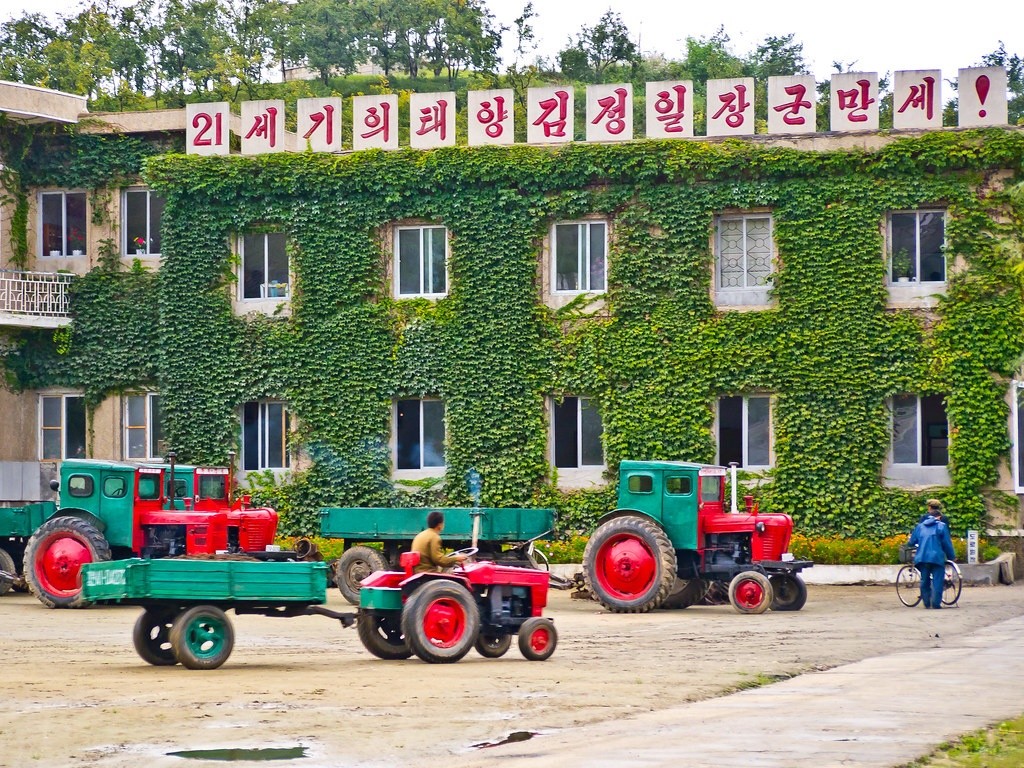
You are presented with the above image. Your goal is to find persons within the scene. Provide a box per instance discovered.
[918,499,950,534]
[410,512,467,573]
[907,511,957,608]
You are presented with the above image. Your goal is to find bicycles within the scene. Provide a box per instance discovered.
[895,543,964,609]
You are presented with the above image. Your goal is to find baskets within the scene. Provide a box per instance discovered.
[899,544,918,561]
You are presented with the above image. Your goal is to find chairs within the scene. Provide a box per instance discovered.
[400,552,421,578]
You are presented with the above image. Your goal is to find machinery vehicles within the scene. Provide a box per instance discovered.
[0,452,229,610]
[80,512,558,670]
[144,450,277,549]
[320,459,814,614]
